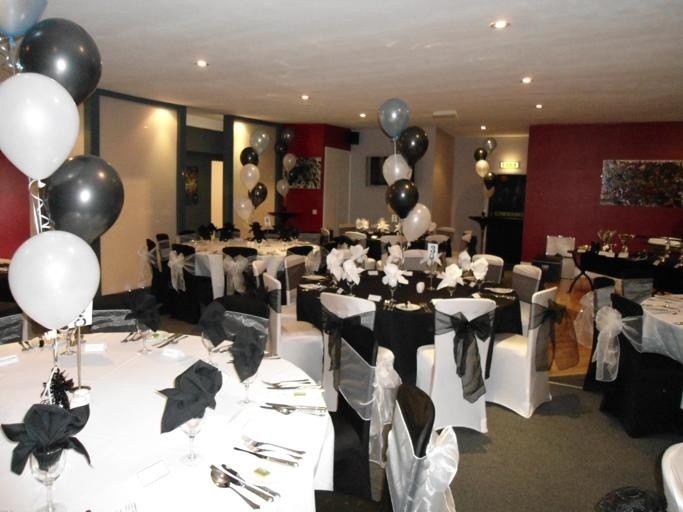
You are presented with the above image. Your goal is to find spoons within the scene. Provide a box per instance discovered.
[211,470,260,510]
[273,405,326,418]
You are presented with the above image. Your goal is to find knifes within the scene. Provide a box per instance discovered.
[210,464,272,501]
[158,334,187,351]
[259,403,332,413]
[267,384,326,393]
[152,331,180,347]
[222,464,280,498]
[234,445,299,468]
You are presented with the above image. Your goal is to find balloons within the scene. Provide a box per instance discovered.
[20,18,106,106]
[474,148,487,161]
[47,153,123,246]
[0,71,79,181]
[377,96,430,243]
[2,0,50,39]
[483,137,496,154]
[233,123,298,232]
[8,230,100,330]
[484,172,496,189]
[476,160,489,178]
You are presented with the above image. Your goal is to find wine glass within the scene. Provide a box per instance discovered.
[348,281,355,296]
[389,279,397,311]
[476,280,484,298]
[29,449,66,512]
[427,264,435,291]
[202,332,218,368]
[61,331,74,357]
[238,373,257,406]
[136,320,151,354]
[179,418,201,463]
[332,275,338,289]
[447,287,456,297]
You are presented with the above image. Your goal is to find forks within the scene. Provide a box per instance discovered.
[243,435,307,455]
[120,326,134,345]
[246,445,303,460]
[262,379,311,386]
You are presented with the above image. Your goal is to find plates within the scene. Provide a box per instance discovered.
[396,303,422,311]
[299,283,327,290]
[490,287,515,293]
[301,274,329,281]
